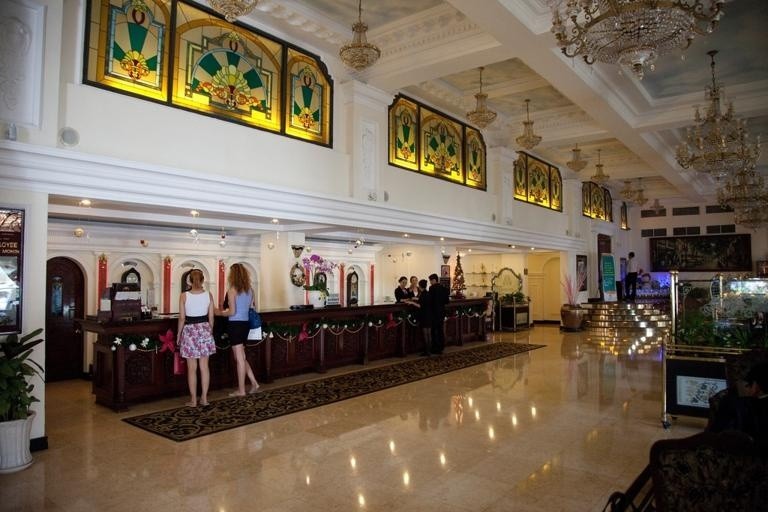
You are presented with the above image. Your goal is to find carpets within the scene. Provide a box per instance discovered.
[121,342,547,442]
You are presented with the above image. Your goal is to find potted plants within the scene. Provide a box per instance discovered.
[503,294,513,305]
[513,292,525,304]
[1,328,45,474]
[560,265,591,332]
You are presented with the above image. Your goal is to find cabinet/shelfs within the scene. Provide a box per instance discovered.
[499,297,529,332]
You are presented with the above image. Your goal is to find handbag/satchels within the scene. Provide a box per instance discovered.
[245,305,263,341]
[173,344,187,376]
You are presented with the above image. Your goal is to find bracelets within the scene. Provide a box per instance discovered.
[177,335,180,337]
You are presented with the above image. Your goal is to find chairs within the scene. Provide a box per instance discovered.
[603,346,767,512]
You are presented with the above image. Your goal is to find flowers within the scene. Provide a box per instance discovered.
[302,254,335,290]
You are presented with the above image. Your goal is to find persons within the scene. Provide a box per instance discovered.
[395,277,412,303]
[404,280,432,357]
[176,269,215,408]
[429,274,449,355]
[223,292,229,310]
[214,263,264,398]
[625,252,640,300]
[407,277,421,301]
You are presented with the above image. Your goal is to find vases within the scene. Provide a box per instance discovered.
[309,289,329,310]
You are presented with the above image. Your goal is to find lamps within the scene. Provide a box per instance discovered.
[567,143,664,214]
[207,0,258,22]
[516,99,542,149]
[673,50,768,232]
[339,0,381,72]
[546,0,726,80]
[466,67,497,129]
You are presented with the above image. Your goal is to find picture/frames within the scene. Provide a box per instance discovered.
[576,255,587,292]
[649,234,752,272]
